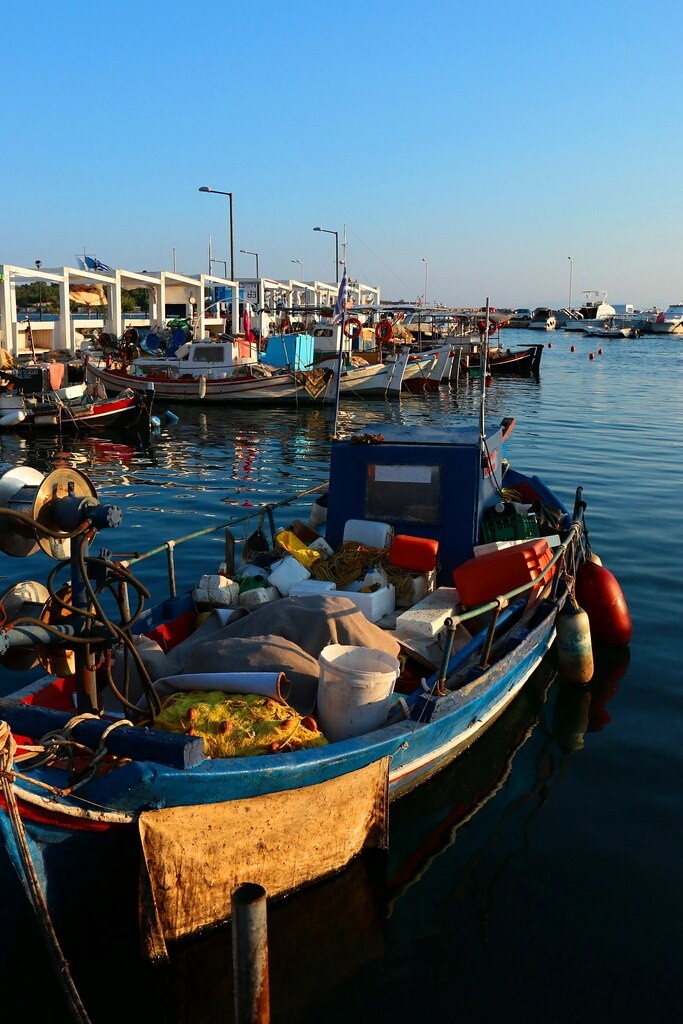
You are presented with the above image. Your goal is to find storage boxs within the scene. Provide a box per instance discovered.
[289,514,557,623]
[50,385,83,400]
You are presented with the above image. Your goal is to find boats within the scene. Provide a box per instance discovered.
[1,296,634,968]
[0,291,683,442]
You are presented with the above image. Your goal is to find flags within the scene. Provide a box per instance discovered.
[334,268,347,314]
[77,256,113,272]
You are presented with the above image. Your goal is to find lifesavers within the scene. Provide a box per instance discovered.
[375,322,394,341]
[344,318,363,340]
[488,318,498,335]
[124,329,140,359]
[398,311,405,322]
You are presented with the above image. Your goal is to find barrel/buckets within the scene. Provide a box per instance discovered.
[162,410,179,420]
[151,416,161,426]
[454,539,557,607]
[343,519,393,554]
[316,643,400,743]
[390,534,439,572]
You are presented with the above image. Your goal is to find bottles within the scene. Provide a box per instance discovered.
[358,569,386,594]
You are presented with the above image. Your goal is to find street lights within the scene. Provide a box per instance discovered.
[568,256,572,308]
[313,227,339,283]
[199,186,235,282]
[240,250,259,303]
[210,260,226,279]
[422,259,428,306]
[291,259,305,283]
